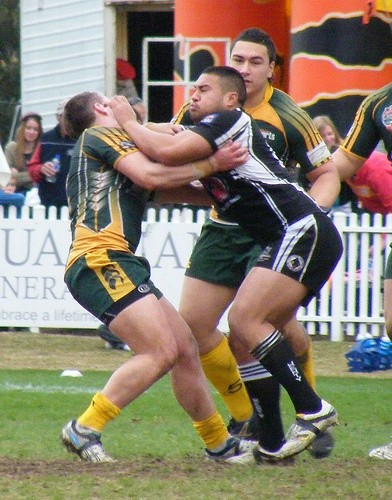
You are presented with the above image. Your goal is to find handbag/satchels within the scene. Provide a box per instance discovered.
[0,190,25,206]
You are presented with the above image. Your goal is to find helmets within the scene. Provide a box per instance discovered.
[22,112,41,121]
[344,337,392,371]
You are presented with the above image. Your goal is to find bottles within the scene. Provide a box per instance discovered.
[45,154,61,183]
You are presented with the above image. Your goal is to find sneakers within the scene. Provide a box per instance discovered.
[275,399,340,457]
[228,415,263,438]
[204,435,262,466]
[226,441,295,467]
[307,429,334,459]
[60,419,113,464]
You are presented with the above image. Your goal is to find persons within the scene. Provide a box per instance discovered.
[312,117,343,154]
[62,91,261,465]
[141,27,340,465]
[112,66,343,467]
[0,111,43,218]
[331,81,392,343]
[116,59,145,122]
[345,150,392,213]
[30,100,77,219]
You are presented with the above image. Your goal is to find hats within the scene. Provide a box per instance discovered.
[116,58,137,80]
[56,97,74,115]
